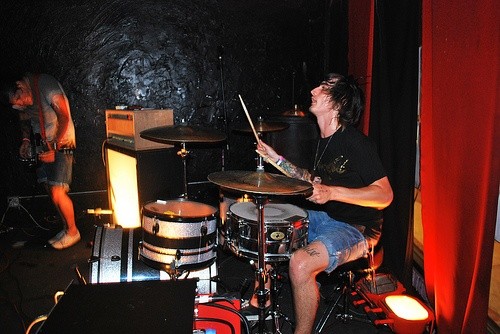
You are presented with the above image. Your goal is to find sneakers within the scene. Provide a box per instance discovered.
[240,298,273,320]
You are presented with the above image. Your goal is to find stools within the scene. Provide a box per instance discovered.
[314,247,378,334]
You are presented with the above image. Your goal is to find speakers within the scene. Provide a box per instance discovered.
[103,142,184,227]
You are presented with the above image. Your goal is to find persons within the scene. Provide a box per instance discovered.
[240,73,394,334]
[6,72,81,249]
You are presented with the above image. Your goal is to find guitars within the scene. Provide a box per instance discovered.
[27,133,76,169]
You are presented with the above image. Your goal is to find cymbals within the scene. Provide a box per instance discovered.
[237,118,293,133]
[264,107,317,124]
[207,170,315,195]
[138,124,228,142]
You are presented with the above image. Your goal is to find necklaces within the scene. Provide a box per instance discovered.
[313,134,333,170]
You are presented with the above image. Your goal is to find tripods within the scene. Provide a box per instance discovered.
[249,264,295,334]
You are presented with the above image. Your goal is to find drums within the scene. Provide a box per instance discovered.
[224,201,311,264]
[217,189,269,248]
[135,198,220,276]
[86,222,218,304]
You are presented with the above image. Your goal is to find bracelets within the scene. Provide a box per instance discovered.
[21,138,31,142]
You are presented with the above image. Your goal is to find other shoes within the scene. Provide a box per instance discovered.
[48,230,81,249]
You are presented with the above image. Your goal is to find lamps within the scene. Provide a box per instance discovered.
[385,294,435,334]
